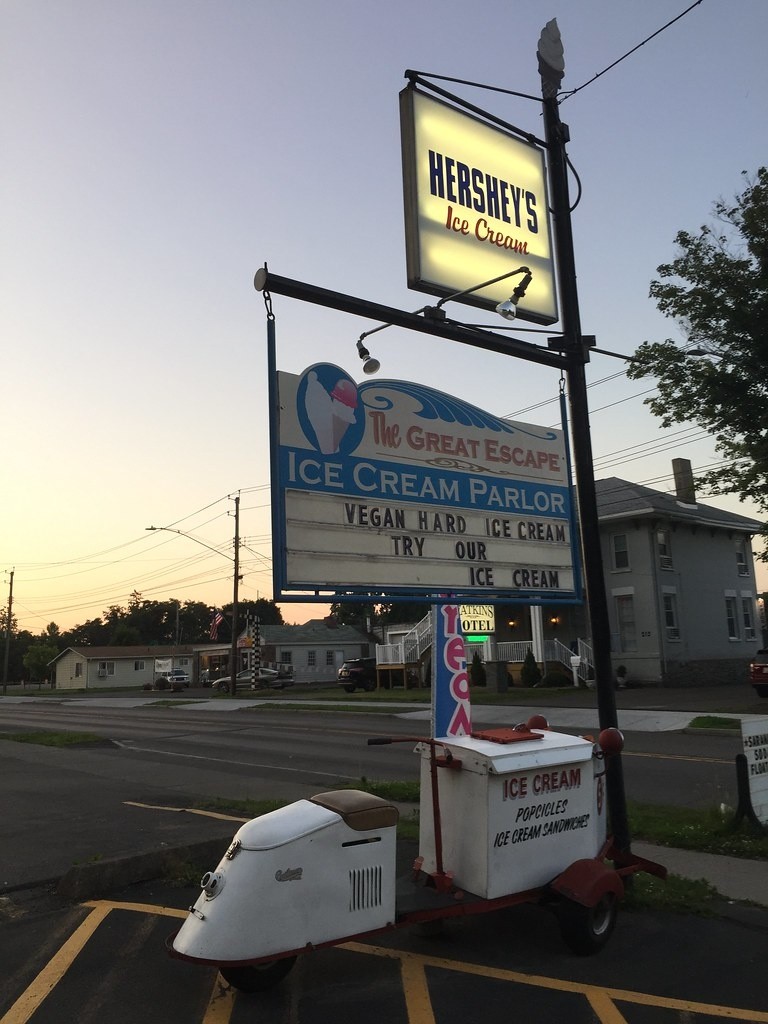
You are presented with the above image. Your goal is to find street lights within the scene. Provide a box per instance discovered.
[144,526,241,697]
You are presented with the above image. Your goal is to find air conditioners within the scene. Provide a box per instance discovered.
[99,669,106,676]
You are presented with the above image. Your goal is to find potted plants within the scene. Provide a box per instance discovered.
[616,665,627,687]
[585,666,596,688]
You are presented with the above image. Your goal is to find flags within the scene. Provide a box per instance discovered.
[208,609,223,641]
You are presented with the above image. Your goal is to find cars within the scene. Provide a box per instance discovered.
[211,666,295,694]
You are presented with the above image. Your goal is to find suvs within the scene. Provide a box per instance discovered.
[199,669,220,688]
[337,657,418,692]
[161,667,190,688]
[749,648,768,699]
